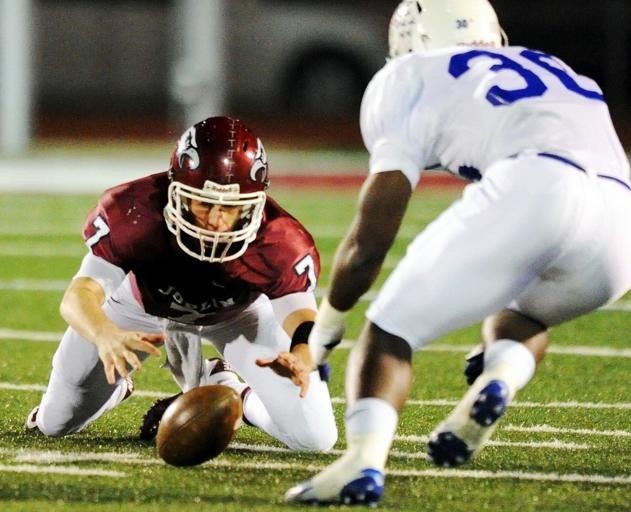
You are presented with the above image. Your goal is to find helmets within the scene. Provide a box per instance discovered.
[164,116,269,263]
[388,0,509,59]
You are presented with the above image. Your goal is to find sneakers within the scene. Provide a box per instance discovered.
[428,373,511,468]
[27,374,135,429]
[139,357,238,441]
[285,456,384,504]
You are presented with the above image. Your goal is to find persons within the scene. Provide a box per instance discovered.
[275,1,631,505]
[24,115,340,458]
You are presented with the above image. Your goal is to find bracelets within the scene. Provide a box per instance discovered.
[290,320,317,352]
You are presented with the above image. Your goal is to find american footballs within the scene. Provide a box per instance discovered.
[157,386,242,466]
